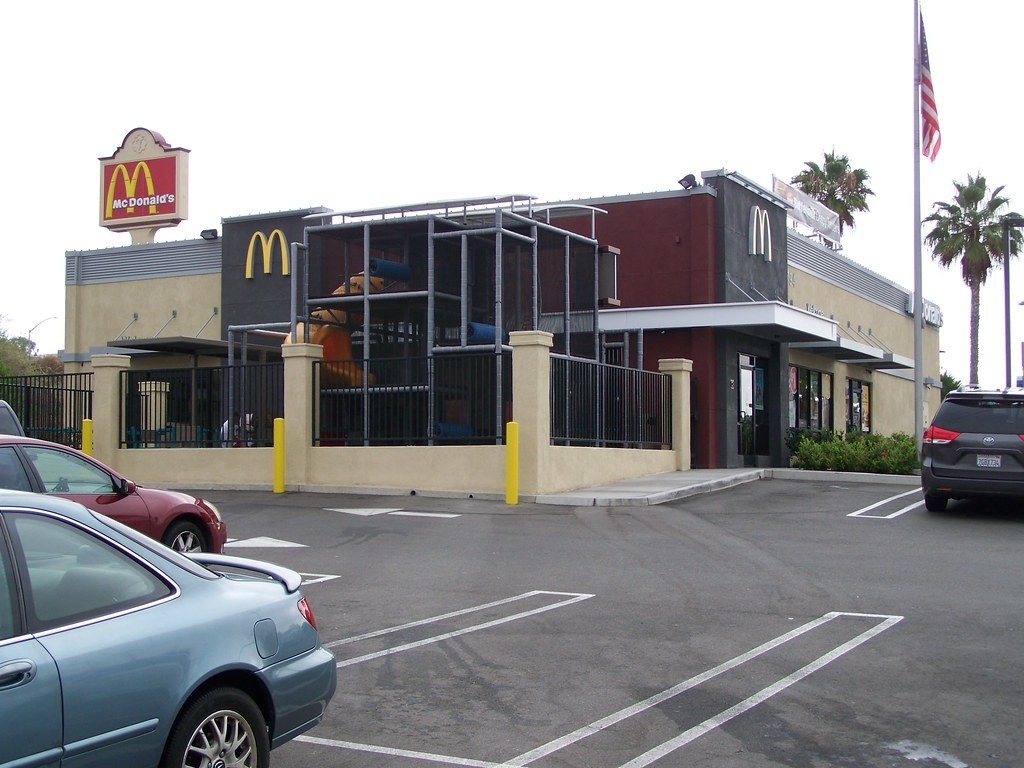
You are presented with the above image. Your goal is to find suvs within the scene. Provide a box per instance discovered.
[920,383,1024,511]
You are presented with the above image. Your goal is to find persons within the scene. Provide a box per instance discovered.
[220,411,242,448]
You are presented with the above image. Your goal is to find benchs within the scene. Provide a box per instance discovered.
[27,567,147,621]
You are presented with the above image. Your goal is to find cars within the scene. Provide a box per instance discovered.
[0,433,227,568]
[0,399,26,437]
[0,488,338,768]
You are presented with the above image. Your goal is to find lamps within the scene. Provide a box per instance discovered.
[678,174,697,189]
[200,229,217,240]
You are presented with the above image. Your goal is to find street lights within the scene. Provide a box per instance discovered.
[28,316,57,360]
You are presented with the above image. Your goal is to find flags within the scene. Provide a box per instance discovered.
[920,6,941,161]
